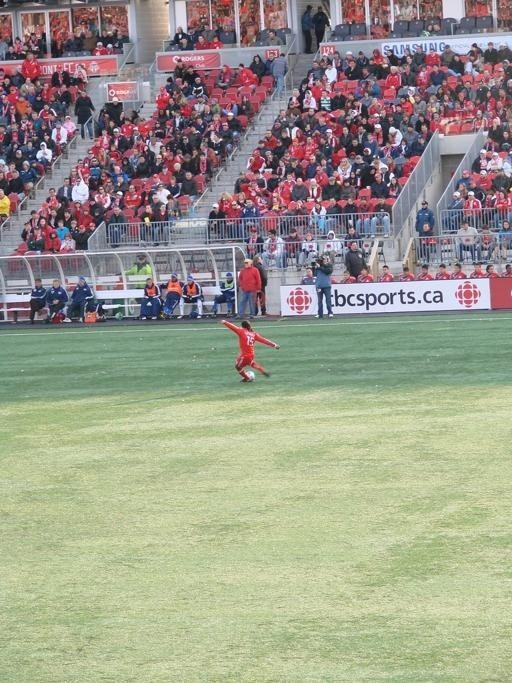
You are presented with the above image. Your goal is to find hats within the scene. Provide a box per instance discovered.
[244,259,253,263]
[171,273,193,281]
[226,272,232,276]
[80,277,86,281]
[136,255,146,259]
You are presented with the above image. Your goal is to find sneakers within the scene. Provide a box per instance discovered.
[315,313,333,318]
[241,378,251,382]
[142,316,157,320]
[63,317,83,323]
[236,311,268,319]
[177,312,234,318]
[28,319,48,323]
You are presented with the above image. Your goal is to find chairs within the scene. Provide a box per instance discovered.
[0,16,512,273]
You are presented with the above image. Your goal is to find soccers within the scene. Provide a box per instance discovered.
[245,371,255,381]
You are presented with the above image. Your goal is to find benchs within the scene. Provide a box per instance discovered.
[0,286,237,322]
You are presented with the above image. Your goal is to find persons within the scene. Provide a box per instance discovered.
[206,272,237,318]
[45,279,68,323]
[140,277,162,319]
[176,274,205,319]
[28,277,47,324]
[300,263,512,285]
[343,241,369,280]
[1,0,512,271]
[220,315,282,384]
[311,250,334,319]
[232,258,262,319]
[115,251,154,320]
[62,275,94,323]
[159,270,185,316]
[252,254,271,316]
